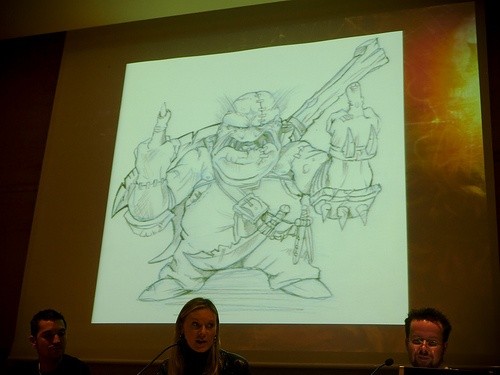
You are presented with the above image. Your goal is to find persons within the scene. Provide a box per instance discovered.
[157,297,248,375]
[19,309,91,375]
[405,307,452,368]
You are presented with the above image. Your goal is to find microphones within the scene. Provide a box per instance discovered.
[138,339,185,375]
[370,358,394,375]
